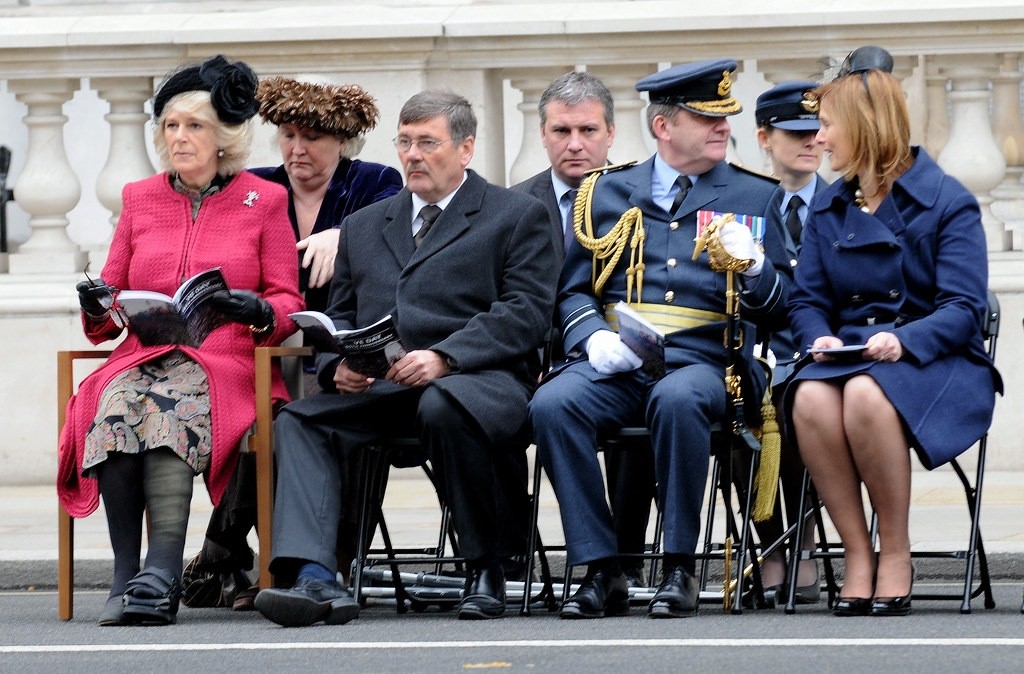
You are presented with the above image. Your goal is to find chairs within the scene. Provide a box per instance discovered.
[58,347,311,620]
[350,289,1001,613]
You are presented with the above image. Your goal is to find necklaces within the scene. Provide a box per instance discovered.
[855,188,869,213]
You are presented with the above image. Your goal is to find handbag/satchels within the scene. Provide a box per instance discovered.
[180,550,224,606]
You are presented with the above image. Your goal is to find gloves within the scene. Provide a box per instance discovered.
[717,221,765,278]
[583,330,644,374]
[75,278,118,317]
[209,287,272,328]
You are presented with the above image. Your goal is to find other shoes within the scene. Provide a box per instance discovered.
[628,571,644,587]
[99,592,125,625]
[120,568,181,619]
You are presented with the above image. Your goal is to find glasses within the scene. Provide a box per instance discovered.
[393,132,463,156]
[84,260,129,329]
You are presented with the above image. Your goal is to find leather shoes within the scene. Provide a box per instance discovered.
[649,565,699,618]
[254,570,361,629]
[233,597,254,613]
[561,575,629,617]
[458,567,505,619]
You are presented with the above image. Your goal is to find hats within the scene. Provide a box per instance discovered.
[154,57,260,125]
[256,76,376,141]
[755,82,824,132]
[637,59,742,118]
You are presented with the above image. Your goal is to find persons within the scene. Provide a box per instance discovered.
[183,78,403,609]
[254,86,560,626]
[505,70,647,588]
[736,79,831,609]
[554,57,788,619]
[57,55,307,628]
[782,47,1008,617]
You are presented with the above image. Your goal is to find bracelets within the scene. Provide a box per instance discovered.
[249,325,269,333]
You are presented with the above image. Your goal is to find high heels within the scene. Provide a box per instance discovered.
[744,572,786,607]
[833,570,877,615]
[871,561,915,615]
[784,560,822,603]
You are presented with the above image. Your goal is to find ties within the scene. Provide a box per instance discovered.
[562,189,575,253]
[787,193,803,244]
[414,205,442,248]
[670,175,690,213]
